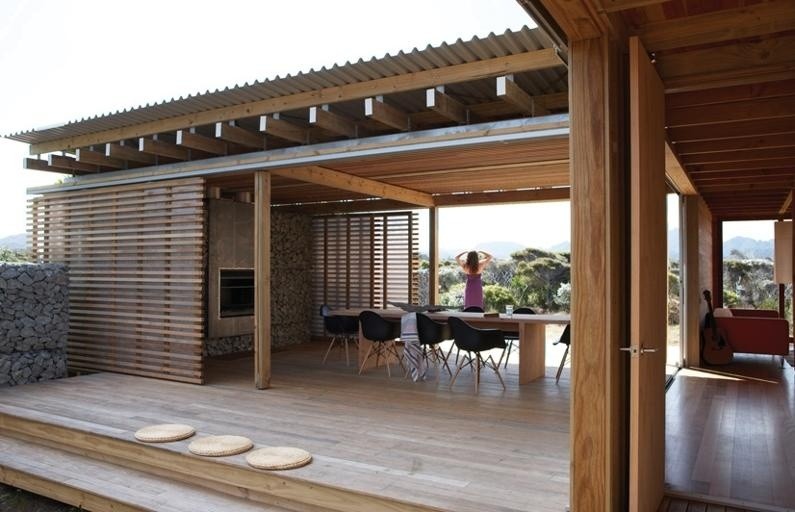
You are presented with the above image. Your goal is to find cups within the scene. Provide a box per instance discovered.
[506,304,513,318]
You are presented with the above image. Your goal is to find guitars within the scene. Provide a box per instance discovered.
[702,289,732,364]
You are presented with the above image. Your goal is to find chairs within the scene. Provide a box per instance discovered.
[320,304,570,394]
[711,303,790,369]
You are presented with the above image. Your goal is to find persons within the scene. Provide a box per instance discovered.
[455,250,494,313]
